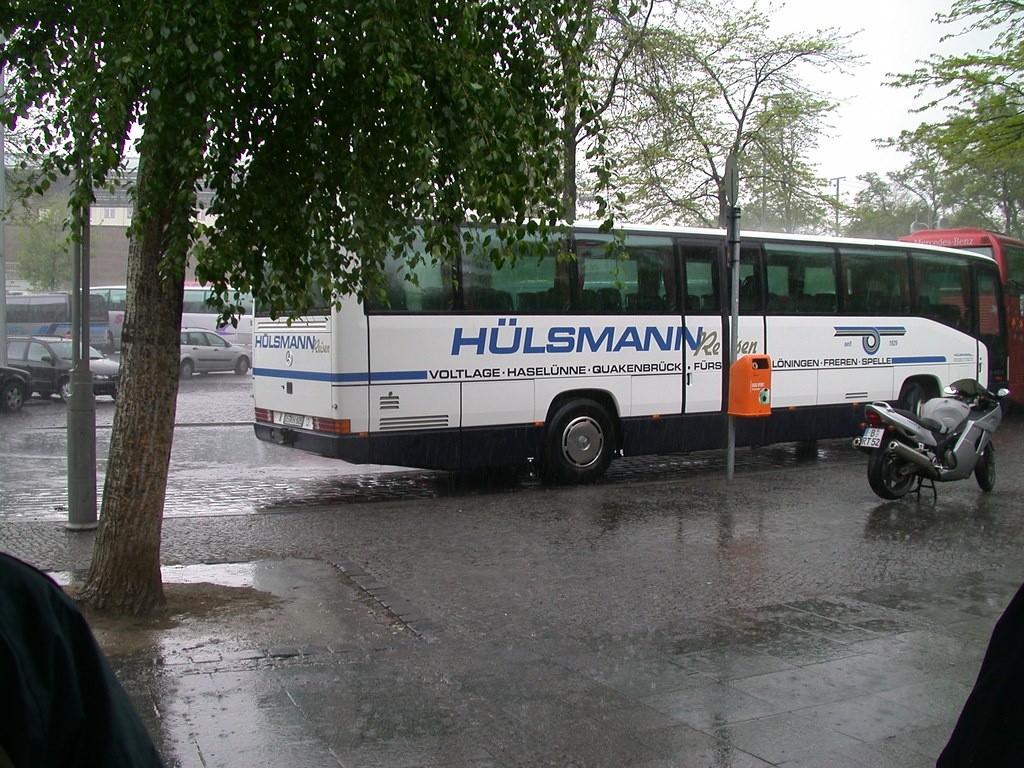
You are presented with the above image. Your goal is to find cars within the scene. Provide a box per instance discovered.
[178,327,252,380]
[5,334,120,403]
[0,364,35,416]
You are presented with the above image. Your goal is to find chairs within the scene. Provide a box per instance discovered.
[191,337,199,345]
[379,285,965,315]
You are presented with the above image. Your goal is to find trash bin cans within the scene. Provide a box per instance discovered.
[727,355,772,417]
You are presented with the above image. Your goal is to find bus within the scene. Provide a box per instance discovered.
[4,290,113,357]
[75,285,255,350]
[252,211,1024,486]
[896,228,1024,413]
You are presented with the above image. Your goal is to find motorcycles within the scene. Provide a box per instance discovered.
[850,378,1011,501]
